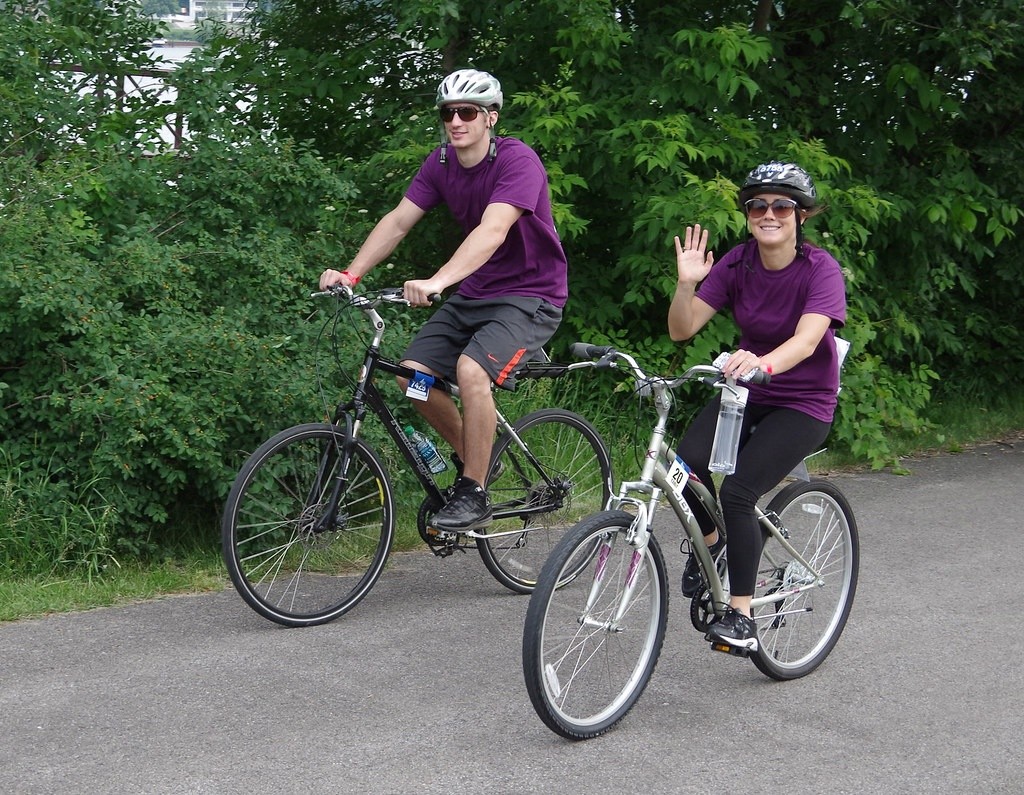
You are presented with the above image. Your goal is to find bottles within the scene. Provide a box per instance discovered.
[404,426,445,473]
[708,375,749,475]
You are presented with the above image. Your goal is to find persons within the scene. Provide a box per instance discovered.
[318,68,568,531]
[667,163,847,655]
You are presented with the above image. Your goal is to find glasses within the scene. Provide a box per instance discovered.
[743,198,801,218]
[437,106,485,122]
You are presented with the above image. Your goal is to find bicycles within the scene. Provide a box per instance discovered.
[219,281,613,629]
[519,345,863,746]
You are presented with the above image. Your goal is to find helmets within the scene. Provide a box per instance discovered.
[740,159,817,210]
[435,69,503,111]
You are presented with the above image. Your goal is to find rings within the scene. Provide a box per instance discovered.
[681,247,689,252]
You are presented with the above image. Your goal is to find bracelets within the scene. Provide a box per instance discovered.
[341,270,360,286]
[757,354,773,375]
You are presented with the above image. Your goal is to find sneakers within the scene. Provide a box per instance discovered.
[681,527,725,598]
[704,608,759,652]
[427,476,493,532]
[450,451,506,491]
